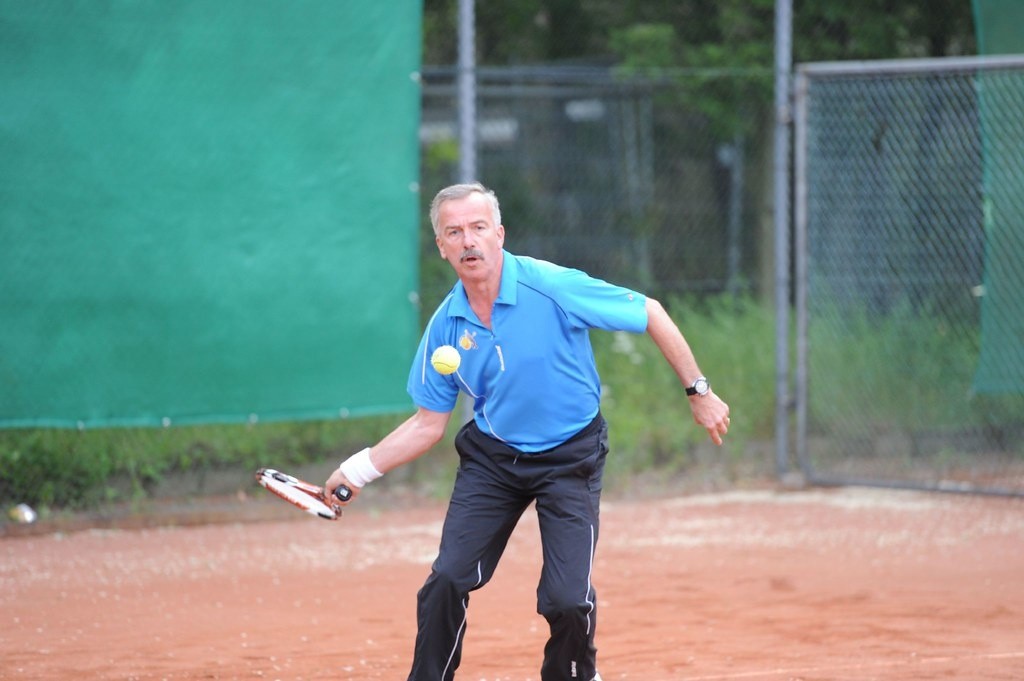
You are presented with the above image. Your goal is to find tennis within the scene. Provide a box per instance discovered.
[431,345,461,375]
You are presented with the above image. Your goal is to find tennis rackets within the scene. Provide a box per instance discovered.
[256,467,351,520]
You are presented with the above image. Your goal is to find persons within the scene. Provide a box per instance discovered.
[330,182,734,681]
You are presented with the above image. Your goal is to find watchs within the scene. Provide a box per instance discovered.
[684,376,710,398]
